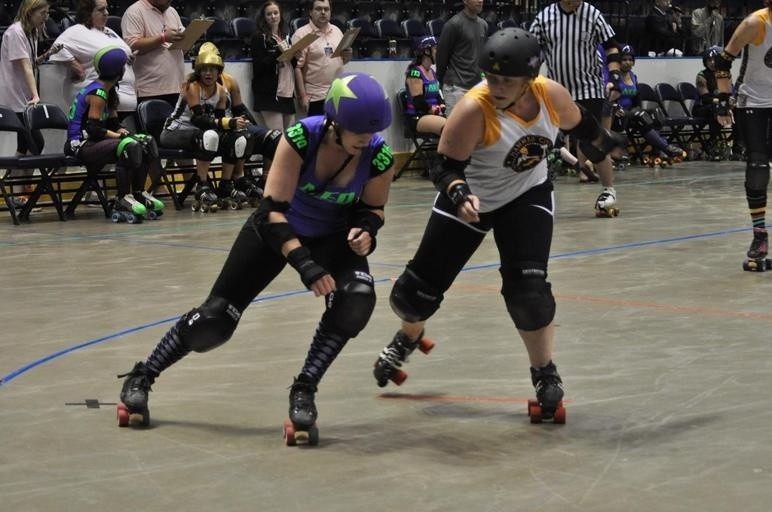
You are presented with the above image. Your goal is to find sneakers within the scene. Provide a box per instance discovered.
[8,195,42,212]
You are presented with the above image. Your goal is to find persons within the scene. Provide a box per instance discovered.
[1,1,282,209]
[710,0,772,261]
[374,26,631,404]
[402,0,772,209]
[253,1,353,128]
[116,71,396,429]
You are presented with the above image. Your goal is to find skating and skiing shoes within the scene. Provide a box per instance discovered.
[711,136,747,161]
[284,373,319,446]
[374,329,434,387]
[595,187,620,218]
[547,148,602,182]
[117,362,156,427]
[640,145,687,167]
[743,228,772,272]
[190,176,266,213]
[610,156,632,171]
[106,190,164,224]
[528,359,565,424]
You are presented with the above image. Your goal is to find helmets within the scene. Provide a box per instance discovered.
[194,42,224,76]
[704,46,724,68]
[478,28,542,77]
[414,36,438,55]
[618,43,635,56]
[324,73,392,133]
[94,47,127,76]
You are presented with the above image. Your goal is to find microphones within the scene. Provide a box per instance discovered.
[668,4,685,15]
[712,7,718,26]
